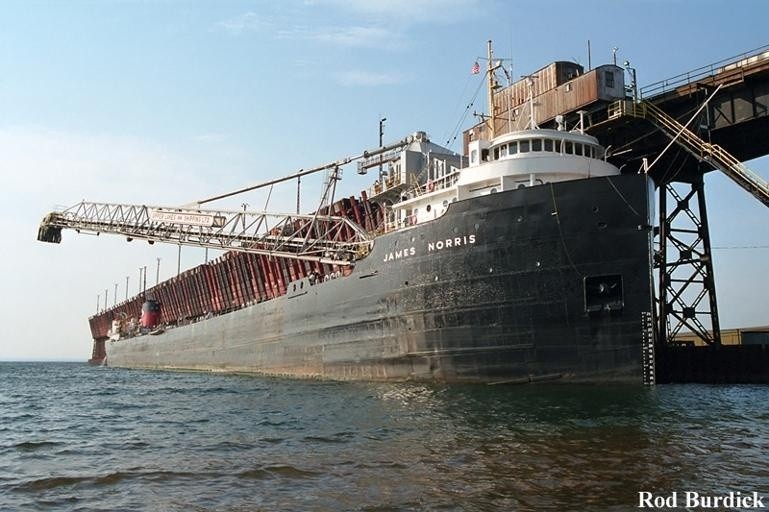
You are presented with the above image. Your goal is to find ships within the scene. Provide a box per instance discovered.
[104,37,657,387]
